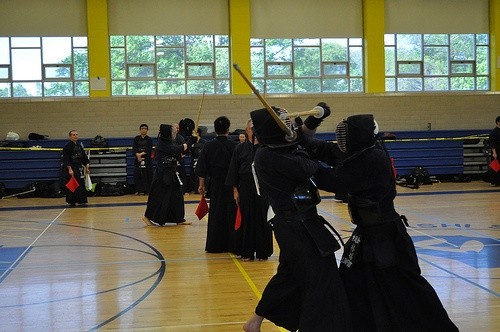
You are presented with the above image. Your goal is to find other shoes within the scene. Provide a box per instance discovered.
[69,203,87,207]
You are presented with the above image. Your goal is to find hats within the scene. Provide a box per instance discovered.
[345,115,375,154]
[250,108,285,137]
[160,124,172,138]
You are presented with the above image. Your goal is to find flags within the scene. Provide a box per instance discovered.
[489,159,500,173]
[195,193,210,221]
[233,202,243,231]
[65,177,79,193]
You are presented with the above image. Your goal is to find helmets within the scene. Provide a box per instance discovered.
[179,118,194,135]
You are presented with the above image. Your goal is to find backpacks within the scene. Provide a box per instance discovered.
[86,181,131,196]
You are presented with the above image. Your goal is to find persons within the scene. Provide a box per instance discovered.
[288,115,459,332]
[489,116,500,187]
[59,129,91,208]
[132,123,156,195]
[143,117,273,262]
[244,102,352,332]
